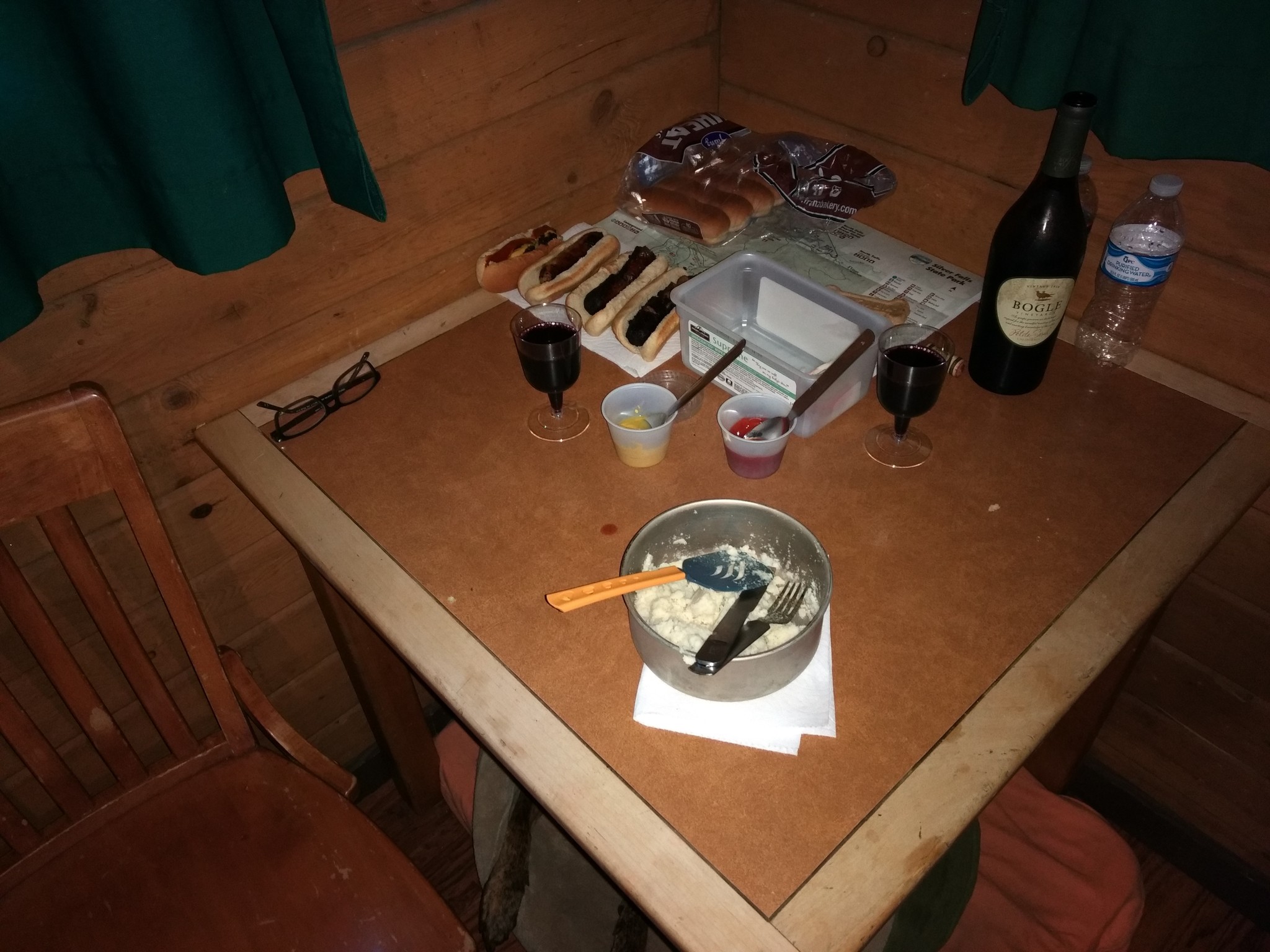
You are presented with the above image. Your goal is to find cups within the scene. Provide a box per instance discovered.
[716,392,798,480]
[601,381,681,469]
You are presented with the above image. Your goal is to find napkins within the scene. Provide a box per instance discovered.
[629,603,839,754]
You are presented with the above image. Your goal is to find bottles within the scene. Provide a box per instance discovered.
[1079,154,1099,231]
[1075,172,1185,374]
[967,87,1096,394]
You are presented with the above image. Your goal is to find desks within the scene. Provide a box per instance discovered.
[191,207,1270,952]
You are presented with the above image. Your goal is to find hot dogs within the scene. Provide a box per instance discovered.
[612,267,695,362]
[476,222,567,293]
[564,245,668,337]
[518,226,620,305]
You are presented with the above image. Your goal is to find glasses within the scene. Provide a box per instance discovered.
[257,351,381,443]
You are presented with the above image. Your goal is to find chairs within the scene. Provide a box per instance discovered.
[0,377,479,952]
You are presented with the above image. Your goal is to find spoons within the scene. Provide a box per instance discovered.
[644,338,746,428]
[743,327,876,443]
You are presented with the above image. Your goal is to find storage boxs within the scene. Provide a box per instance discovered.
[673,251,890,439]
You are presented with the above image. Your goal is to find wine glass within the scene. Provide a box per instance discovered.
[510,302,592,441]
[862,323,957,468]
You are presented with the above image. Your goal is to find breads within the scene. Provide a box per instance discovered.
[824,285,911,328]
[626,149,788,244]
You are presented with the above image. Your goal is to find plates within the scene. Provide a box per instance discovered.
[639,369,705,424]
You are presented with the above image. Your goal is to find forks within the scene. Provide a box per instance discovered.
[691,578,813,678]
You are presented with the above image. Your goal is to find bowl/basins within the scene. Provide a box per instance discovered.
[617,499,835,703]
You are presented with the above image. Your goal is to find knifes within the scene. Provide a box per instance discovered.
[695,564,776,667]
